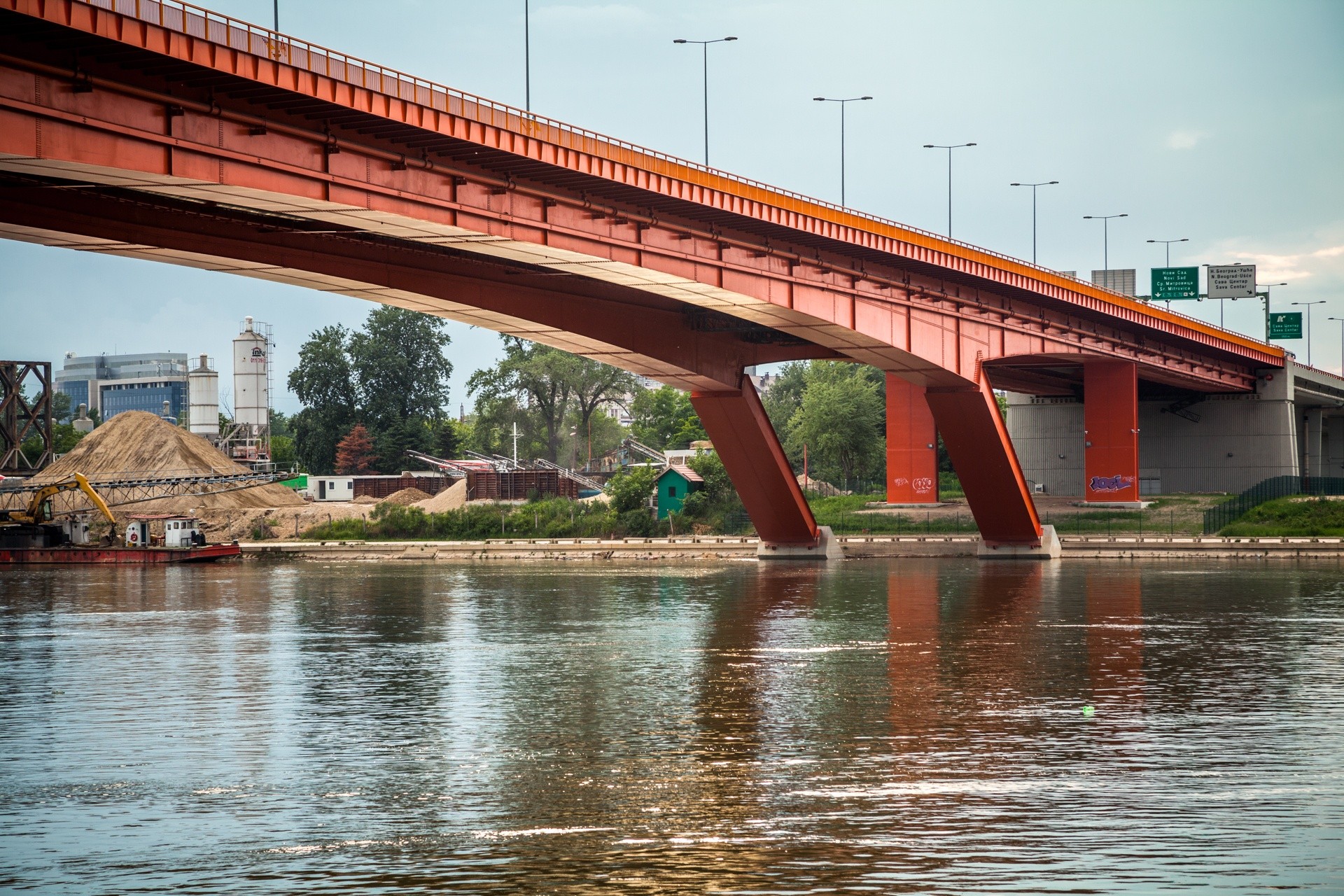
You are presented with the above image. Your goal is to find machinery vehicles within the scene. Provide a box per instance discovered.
[2,473,126,548]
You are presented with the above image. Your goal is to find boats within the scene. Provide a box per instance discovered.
[2,515,244,567]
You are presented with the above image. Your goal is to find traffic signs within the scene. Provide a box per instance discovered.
[1206,264,1257,300]
[1270,312,1305,340]
[1150,266,1201,300]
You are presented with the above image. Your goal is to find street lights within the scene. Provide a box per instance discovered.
[1082,214,1133,290]
[1145,239,1191,312]
[1252,283,1288,344]
[673,35,737,174]
[923,143,981,243]
[1328,317,1344,379]
[813,97,875,212]
[1292,300,1327,371]
[1203,262,1245,329]
[1010,181,1060,270]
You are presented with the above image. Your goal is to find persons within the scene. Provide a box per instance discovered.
[608,459,612,471]
[1142,295,1149,305]
[199,530,205,546]
